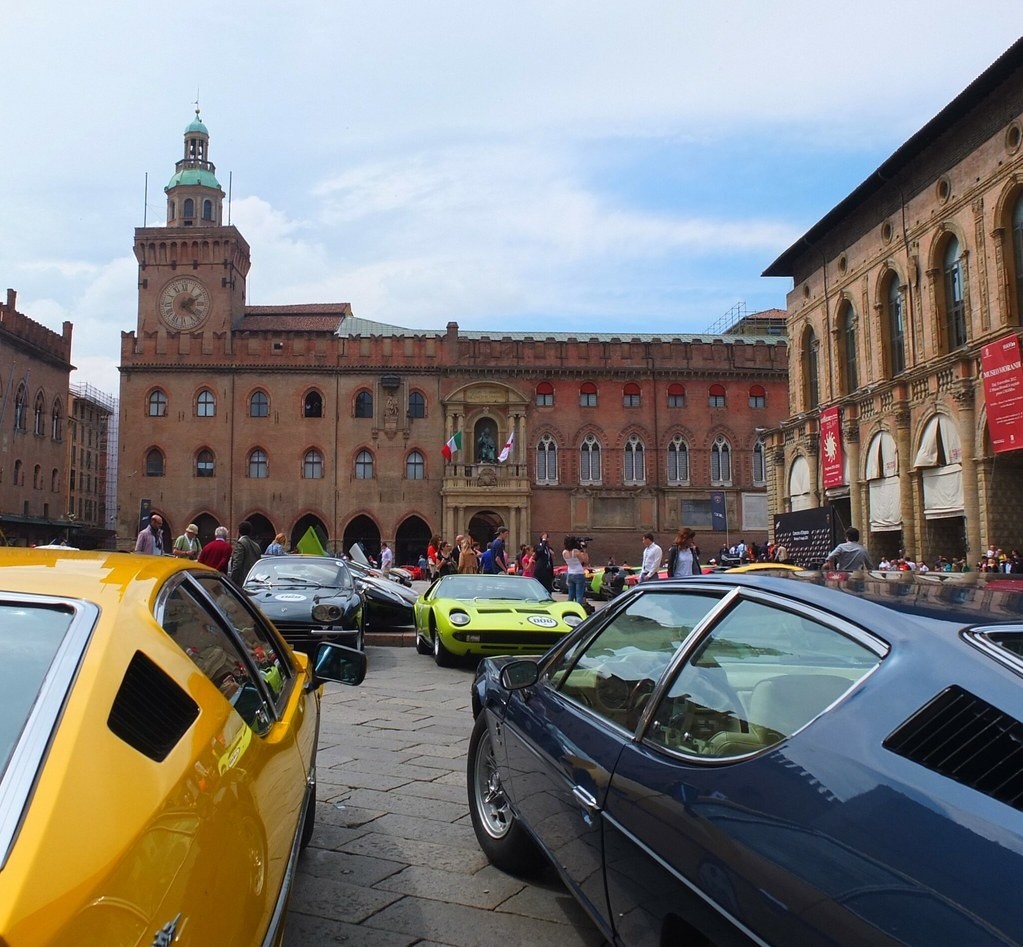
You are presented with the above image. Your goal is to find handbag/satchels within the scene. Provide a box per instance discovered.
[742,545,748,559]
[378,553,382,563]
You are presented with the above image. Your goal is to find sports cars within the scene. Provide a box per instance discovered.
[412,573,587,667]
[0,544,372,947]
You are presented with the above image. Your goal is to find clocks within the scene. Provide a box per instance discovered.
[160,278,210,329]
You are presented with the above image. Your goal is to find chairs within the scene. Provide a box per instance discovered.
[700,671,852,761]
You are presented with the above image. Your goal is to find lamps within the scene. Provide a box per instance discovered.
[798,412,806,420]
[867,384,877,394]
[755,427,765,433]
[780,421,788,428]
[818,406,826,413]
[925,363,936,368]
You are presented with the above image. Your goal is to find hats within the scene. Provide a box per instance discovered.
[494,526,510,534]
[215,526,228,537]
[419,555,423,558]
[989,545,996,548]
[987,559,995,566]
[998,549,1002,551]
[186,524,198,534]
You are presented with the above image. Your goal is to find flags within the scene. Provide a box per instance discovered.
[497,432,515,463]
[441,431,461,462]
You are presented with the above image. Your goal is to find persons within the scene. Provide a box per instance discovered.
[976,544,1023,574]
[822,528,873,572]
[719,539,787,566]
[369,542,392,575]
[597,557,628,600]
[639,533,663,583]
[136,515,164,556]
[478,428,495,464]
[935,555,968,572]
[418,555,427,573]
[562,534,589,606]
[427,527,509,584]
[515,532,554,597]
[231,522,262,587]
[198,526,235,575]
[173,524,202,561]
[264,532,287,555]
[668,528,701,578]
[879,556,929,572]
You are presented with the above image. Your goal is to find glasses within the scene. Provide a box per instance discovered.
[380,545,383,547]
[455,539,459,541]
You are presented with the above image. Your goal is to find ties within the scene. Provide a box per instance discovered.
[545,548,550,566]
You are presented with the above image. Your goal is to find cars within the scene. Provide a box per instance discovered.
[552,564,806,601]
[464,566,1023,947]
[275,563,420,627]
[240,555,366,655]
